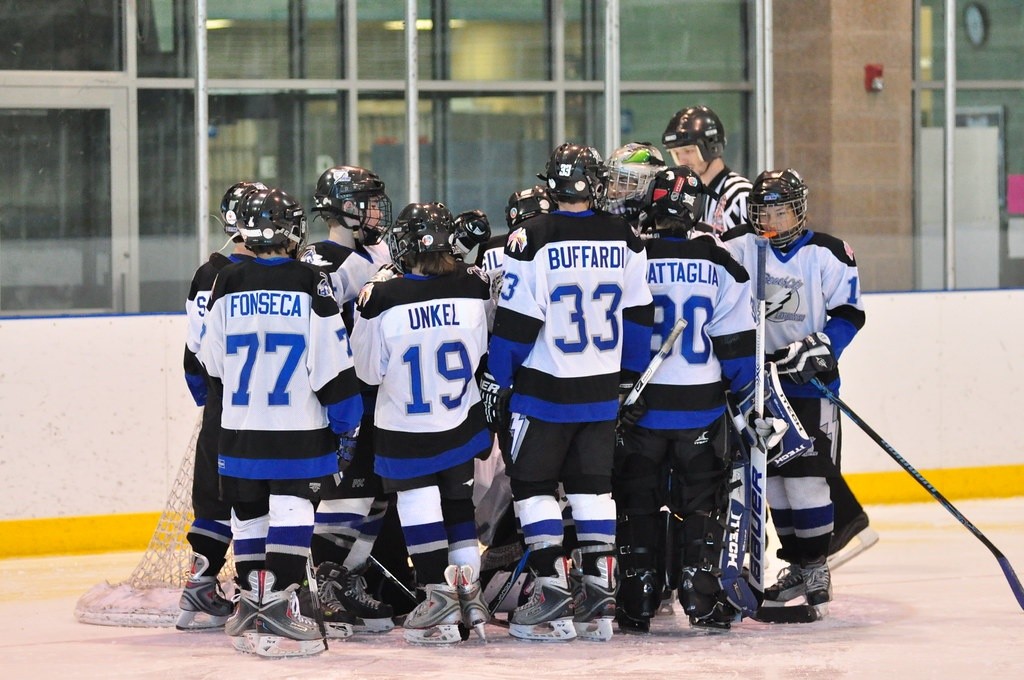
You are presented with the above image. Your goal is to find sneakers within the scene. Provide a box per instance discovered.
[401,506,879,647]
[297,561,356,639]
[176,552,235,630]
[226,570,261,653]
[254,569,326,657]
[339,560,395,632]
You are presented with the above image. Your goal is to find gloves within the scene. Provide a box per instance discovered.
[479,370,515,424]
[736,378,789,450]
[337,420,361,466]
[614,377,647,428]
[450,209,491,254]
[769,331,839,384]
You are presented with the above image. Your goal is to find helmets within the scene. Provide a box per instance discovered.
[745,168,808,248]
[388,201,455,275]
[505,186,559,231]
[236,189,309,261]
[313,166,393,247]
[603,142,669,218]
[219,181,269,234]
[646,165,706,230]
[661,104,726,162]
[546,143,609,212]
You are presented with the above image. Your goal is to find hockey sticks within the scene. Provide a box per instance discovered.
[748,235,818,624]
[808,374,1024,612]
[487,318,690,630]
[305,554,329,651]
[367,554,421,605]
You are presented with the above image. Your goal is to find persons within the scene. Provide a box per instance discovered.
[177,104,879,657]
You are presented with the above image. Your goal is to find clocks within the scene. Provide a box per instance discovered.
[963,3,987,48]
